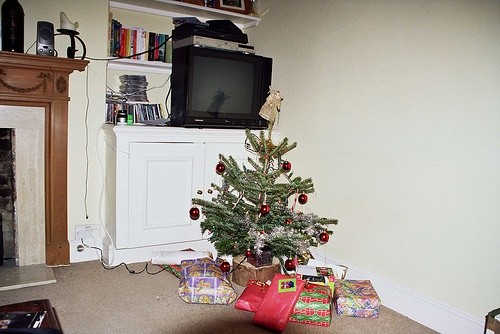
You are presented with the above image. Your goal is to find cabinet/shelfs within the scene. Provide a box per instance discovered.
[102,0,279,269]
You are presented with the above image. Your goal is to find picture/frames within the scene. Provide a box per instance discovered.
[215,0,251,15]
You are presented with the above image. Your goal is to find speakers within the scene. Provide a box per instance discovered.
[36,22,54,56]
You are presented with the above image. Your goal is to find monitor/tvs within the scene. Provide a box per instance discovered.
[172,43,272,128]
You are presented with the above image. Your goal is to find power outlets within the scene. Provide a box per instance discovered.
[76,224,100,240]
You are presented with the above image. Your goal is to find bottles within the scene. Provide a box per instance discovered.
[116,110,127,123]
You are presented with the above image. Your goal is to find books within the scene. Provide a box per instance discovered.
[108,11,173,63]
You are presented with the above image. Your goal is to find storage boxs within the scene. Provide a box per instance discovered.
[333,280,381,319]
[290,286,332,326]
